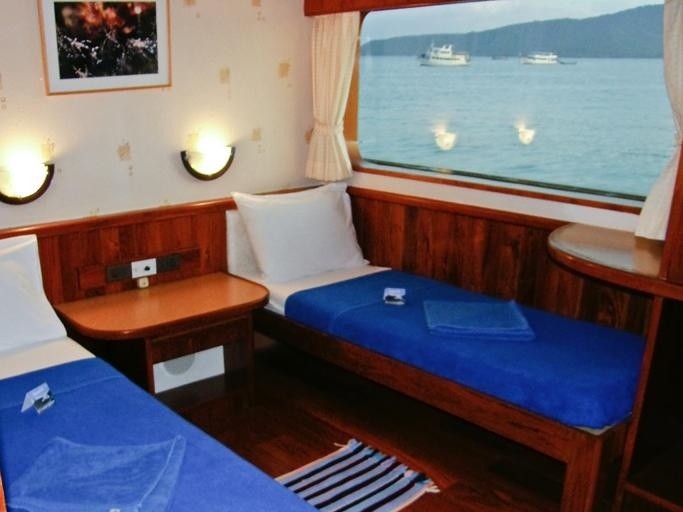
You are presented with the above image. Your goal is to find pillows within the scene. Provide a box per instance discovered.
[0,241,68,358]
[230,183,370,285]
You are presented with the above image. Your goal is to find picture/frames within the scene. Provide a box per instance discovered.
[34,2,175,94]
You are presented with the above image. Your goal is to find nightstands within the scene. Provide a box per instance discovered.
[56,269,272,422]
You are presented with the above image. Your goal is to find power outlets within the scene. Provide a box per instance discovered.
[131,258,157,279]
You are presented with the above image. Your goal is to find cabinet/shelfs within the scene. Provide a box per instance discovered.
[546,212,680,508]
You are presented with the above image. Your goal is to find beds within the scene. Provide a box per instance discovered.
[0,231,318,509]
[227,180,643,506]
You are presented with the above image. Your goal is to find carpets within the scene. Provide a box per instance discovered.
[274,437,441,512]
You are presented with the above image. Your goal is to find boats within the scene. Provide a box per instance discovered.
[522,52,557,64]
[419,43,471,65]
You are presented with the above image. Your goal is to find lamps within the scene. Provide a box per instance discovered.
[179,129,239,181]
[0,139,56,205]
